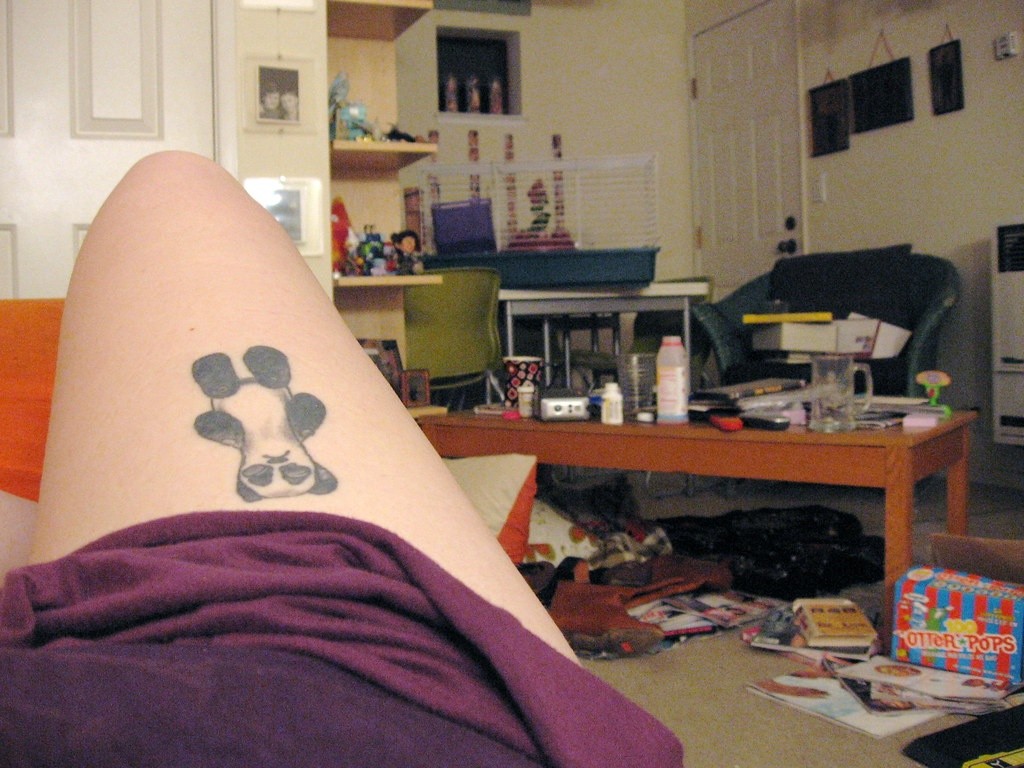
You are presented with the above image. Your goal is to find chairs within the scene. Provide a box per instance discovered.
[403,266,507,406]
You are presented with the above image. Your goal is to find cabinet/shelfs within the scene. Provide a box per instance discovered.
[328,0,444,290]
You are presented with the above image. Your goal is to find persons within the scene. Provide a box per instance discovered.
[0,149,684,768]
[259,81,297,119]
[390,230,420,276]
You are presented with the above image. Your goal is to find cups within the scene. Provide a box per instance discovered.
[616,352,657,422]
[503,357,545,418]
[807,355,873,433]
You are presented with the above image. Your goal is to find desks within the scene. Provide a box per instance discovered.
[497,281,711,400]
[420,396,978,648]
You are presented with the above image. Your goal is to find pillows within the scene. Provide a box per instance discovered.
[752,243,912,395]
[436,453,539,563]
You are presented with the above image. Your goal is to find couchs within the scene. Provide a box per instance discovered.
[694,242,960,502]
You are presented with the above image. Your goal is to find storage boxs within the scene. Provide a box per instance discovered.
[418,242,661,289]
[892,533,1024,686]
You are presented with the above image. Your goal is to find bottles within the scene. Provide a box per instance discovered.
[601,382,624,425]
[656,336,690,424]
[516,386,534,417]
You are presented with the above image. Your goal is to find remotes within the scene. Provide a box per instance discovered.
[741,414,792,431]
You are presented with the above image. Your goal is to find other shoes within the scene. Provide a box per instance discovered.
[548,583,665,657]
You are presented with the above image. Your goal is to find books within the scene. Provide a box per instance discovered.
[627,588,878,661]
[473,403,503,415]
[695,377,806,399]
